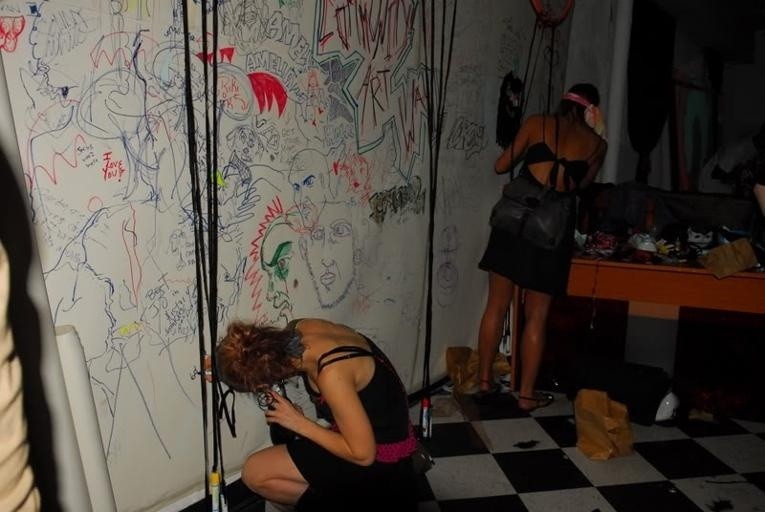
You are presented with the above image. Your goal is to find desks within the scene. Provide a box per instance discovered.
[507,258,764,392]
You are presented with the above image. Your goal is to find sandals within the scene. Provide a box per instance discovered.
[518,391,554,413]
[471,382,502,401]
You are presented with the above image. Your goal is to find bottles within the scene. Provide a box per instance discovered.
[420,399,433,439]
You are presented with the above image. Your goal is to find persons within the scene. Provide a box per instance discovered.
[470,78,609,413]
[200,313,420,510]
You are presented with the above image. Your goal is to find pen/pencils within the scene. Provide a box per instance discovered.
[197,371,211,375]
